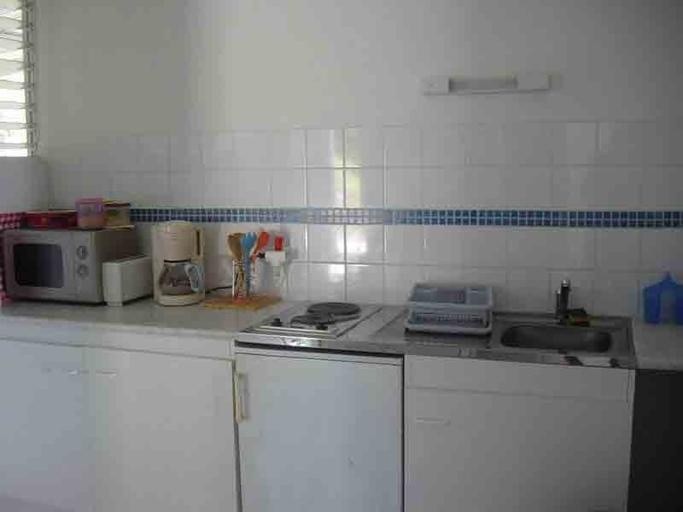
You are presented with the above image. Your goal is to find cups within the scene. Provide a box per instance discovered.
[231,257,257,299]
[74,197,131,229]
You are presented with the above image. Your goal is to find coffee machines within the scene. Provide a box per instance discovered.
[150,219,207,308]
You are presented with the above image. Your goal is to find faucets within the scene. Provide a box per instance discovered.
[557,277,571,322]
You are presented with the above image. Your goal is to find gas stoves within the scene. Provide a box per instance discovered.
[233,295,384,351]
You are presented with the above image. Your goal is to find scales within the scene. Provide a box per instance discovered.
[404,285,495,337]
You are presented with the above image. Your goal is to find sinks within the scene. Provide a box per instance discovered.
[500,321,614,355]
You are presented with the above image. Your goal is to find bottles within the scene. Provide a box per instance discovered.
[641,271,681,323]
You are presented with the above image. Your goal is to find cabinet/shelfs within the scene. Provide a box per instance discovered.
[0,318,242,512]
[403,358,634,511]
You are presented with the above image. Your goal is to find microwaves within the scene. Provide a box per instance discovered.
[2,225,137,305]
